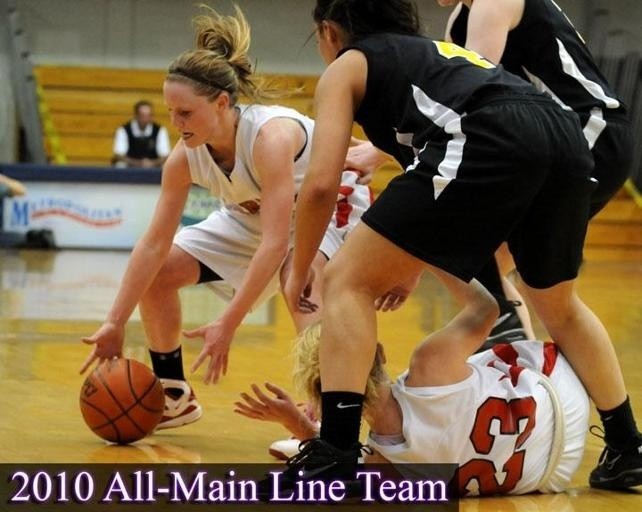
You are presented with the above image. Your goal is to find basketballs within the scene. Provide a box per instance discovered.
[79,357,165,445]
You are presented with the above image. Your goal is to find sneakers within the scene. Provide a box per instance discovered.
[269,421,369,464]
[589,425,642,490]
[153,378,202,431]
[256,437,373,504]
[475,301,528,354]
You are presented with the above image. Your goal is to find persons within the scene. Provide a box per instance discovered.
[80,14,380,462]
[342,0,634,353]
[111,100,172,170]
[235,266,592,497]
[0,174,26,235]
[246,0,642,501]
